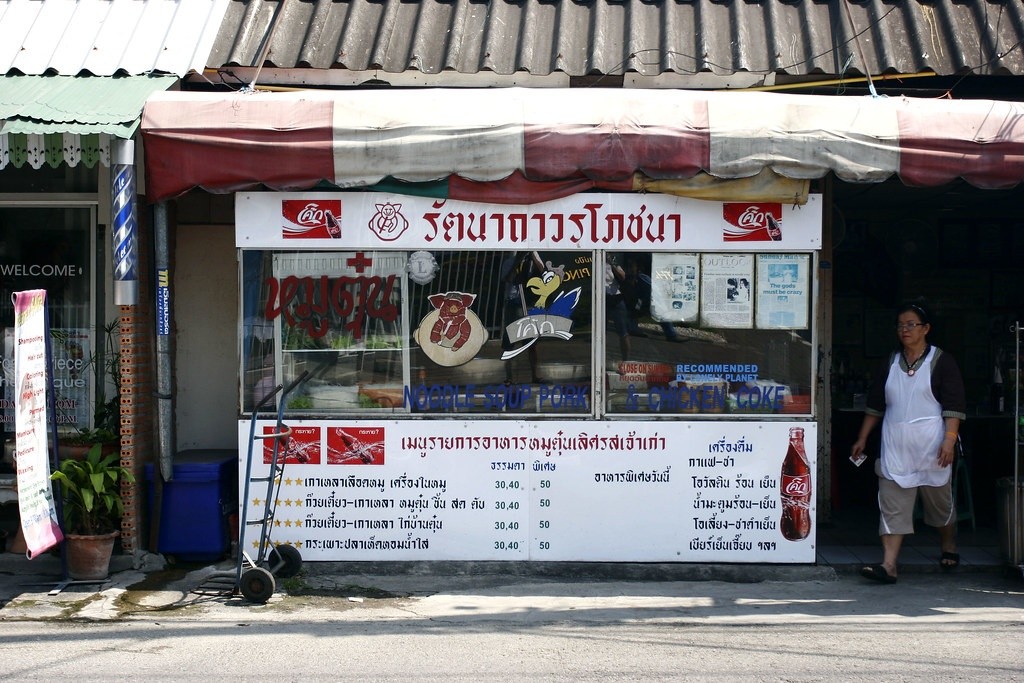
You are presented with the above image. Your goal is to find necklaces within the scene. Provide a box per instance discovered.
[903,345,927,377]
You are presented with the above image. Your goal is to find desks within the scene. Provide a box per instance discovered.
[835,400,1012,535]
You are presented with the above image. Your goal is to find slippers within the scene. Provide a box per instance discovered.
[859,563,898,586]
[940,550,960,567]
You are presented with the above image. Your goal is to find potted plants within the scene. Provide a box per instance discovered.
[57,427,121,460]
[52,444,136,580]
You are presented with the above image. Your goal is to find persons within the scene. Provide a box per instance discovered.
[728,278,749,301]
[501,251,554,387]
[605,252,689,376]
[852,304,965,583]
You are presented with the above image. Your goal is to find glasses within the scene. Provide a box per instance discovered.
[896,321,925,332]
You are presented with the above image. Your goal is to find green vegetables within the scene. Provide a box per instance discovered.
[282,310,400,409]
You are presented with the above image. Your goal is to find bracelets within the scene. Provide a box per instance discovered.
[945,431,958,442]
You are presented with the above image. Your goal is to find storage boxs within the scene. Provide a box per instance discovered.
[143,448,238,563]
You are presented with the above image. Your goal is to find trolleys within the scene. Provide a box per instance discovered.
[186,372,305,606]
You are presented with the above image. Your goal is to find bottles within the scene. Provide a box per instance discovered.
[766,212,782,241]
[336,428,374,464]
[780,427,811,541]
[272,428,309,464]
[416,367,429,412]
[324,210,341,238]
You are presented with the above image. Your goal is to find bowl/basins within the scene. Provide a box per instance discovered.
[678,380,725,413]
[448,357,506,385]
[536,362,587,383]
[615,397,659,412]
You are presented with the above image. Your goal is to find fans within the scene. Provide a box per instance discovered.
[832,202,845,251]
[883,219,940,274]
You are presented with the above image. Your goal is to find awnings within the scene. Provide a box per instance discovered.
[140,87,1024,204]
[0,73,181,140]
